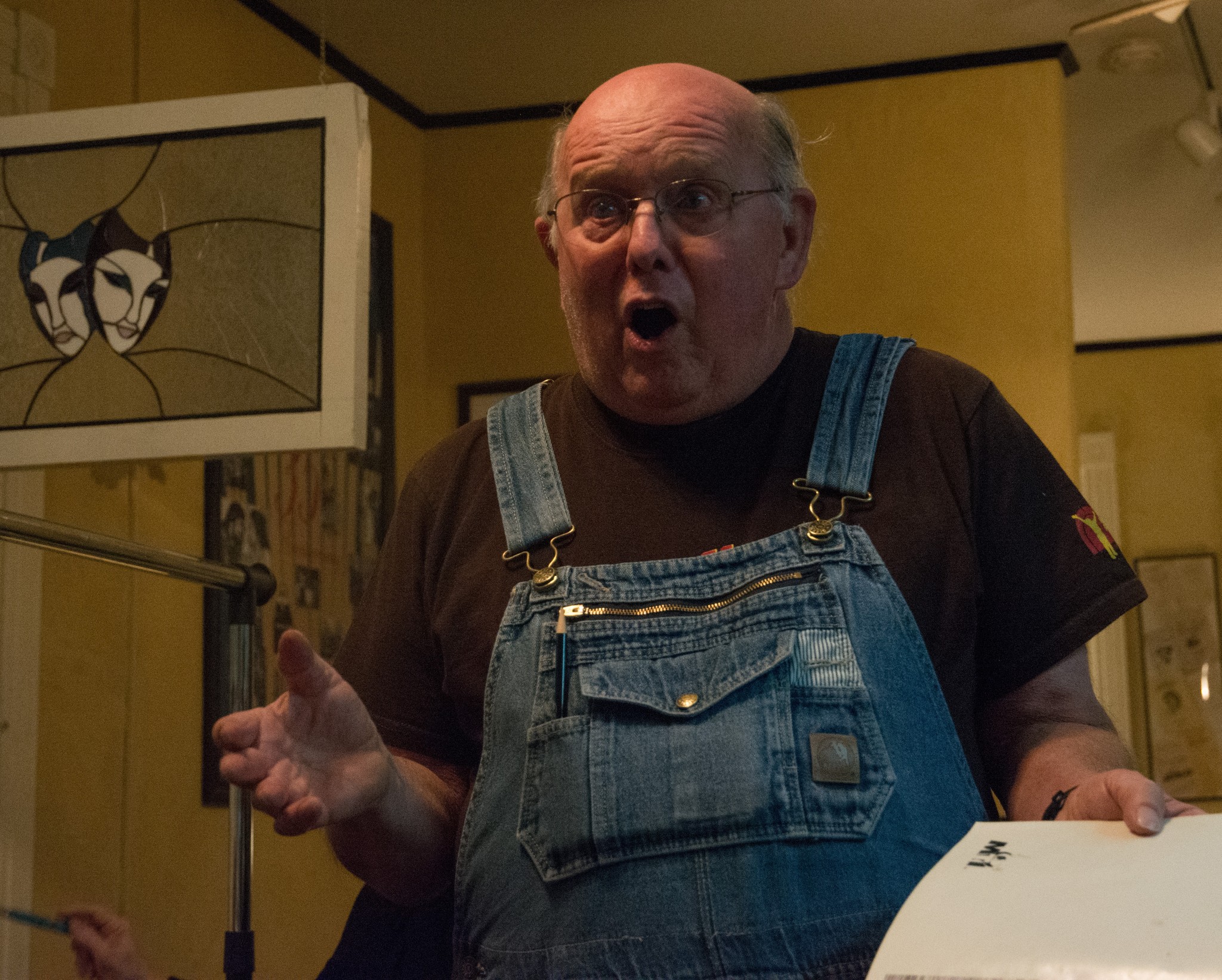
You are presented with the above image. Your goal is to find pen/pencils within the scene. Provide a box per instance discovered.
[0,906,69,936]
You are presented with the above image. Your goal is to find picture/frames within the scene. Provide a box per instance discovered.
[1132,550,1222,803]
[454,373,554,435]
[197,205,397,811]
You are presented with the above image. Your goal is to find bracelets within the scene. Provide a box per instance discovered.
[1040,784,1079,822]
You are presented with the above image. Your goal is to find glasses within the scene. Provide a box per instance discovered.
[546,177,791,247]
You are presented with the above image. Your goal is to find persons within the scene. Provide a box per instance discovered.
[57,902,170,979]
[217,60,1208,980]
